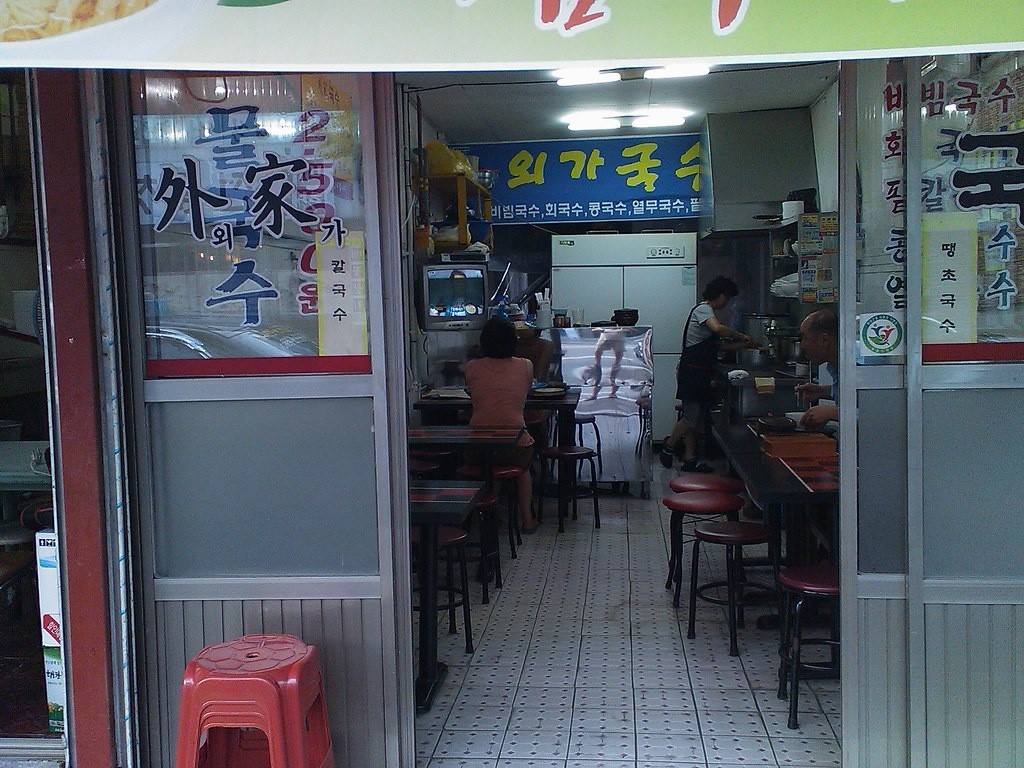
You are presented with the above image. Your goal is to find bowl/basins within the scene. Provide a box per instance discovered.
[786,412,821,432]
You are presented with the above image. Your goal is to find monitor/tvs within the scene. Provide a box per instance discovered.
[417,263,488,331]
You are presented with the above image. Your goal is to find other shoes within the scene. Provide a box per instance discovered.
[583,396,597,401]
[609,393,617,398]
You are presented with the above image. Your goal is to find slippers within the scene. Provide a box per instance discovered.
[660,436,675,468]
[681,458,714,473]
[522,519,541,534]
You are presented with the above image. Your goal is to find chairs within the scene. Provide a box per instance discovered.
[635,396,652,458]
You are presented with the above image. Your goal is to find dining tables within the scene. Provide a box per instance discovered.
[711,421,840,669]
[406,424,526,586]
[408,476,487,714]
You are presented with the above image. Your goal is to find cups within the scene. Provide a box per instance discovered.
[794,363,808,375]
[572,308,584,327]
[510,304,519,314]
[536,310,551,329]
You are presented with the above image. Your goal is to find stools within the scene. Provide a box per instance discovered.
[405,415,604,652]
[663,470,841,730]
[177,632,335,768]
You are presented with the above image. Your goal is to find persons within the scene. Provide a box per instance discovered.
[585,326,632,400]
[794,309,839,429]
[508,327,555,478]
[437,270,482,317]
[660,276,759,472]
[462,317,539,533]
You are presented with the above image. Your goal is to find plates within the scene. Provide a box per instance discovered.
[536,387,564,393]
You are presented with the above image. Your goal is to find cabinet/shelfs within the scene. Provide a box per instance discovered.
[768,210,841,304]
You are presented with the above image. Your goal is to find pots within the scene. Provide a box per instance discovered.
[736,347,769,369]
[784,337,805,361]
[735,311,791,343]
